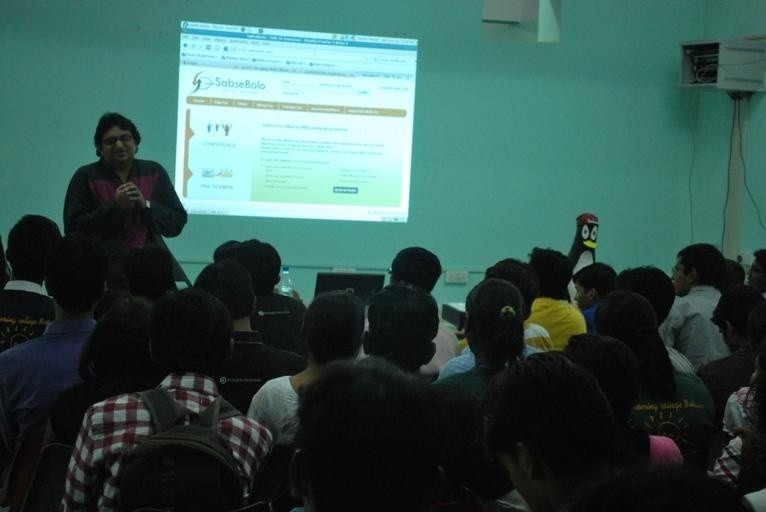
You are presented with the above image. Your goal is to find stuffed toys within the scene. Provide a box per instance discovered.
[566,212,599,278]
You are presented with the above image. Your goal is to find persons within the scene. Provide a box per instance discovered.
[194,259,305,413]
[655,240,726,372]
[207,239,308,334]
[692,287,766,418]
[593,289,716,441]
[0,237,109,455]
[54,289,274,512]
[522,244,586,352]
[60,112,192,301]
[357,287,440,371]
[455,255,552,356]
[575,260,617,336]
[243,290,364,501]
[390,245,460,373]
[51,296,158,500]
[747,248,765,288]
[427,279,536,497]
[719,258,744,293]
[558,335,685,466]
[704,351,764,498]
[1,214,58,352]
[611,262,710,410]
[486,355,752,511]
[286,358,485,511]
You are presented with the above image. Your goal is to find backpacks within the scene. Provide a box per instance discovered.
[112,392,262,512]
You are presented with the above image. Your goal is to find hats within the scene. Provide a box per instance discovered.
[213,240,281,287]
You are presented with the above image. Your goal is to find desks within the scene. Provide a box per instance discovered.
[442,301,466,331]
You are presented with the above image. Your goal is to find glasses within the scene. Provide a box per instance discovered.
[751,264,763,273]
[98,133,137,146]
[387,267,401,282]
[667,265,690,277]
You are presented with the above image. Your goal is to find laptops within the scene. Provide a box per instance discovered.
[314,272,385,303]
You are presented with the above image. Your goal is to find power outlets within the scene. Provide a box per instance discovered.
[446,271,468,283]
[737,254,755,265]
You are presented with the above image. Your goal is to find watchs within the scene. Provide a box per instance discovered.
[144,198,151,212]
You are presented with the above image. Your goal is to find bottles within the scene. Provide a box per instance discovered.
[278,266,293,297]
